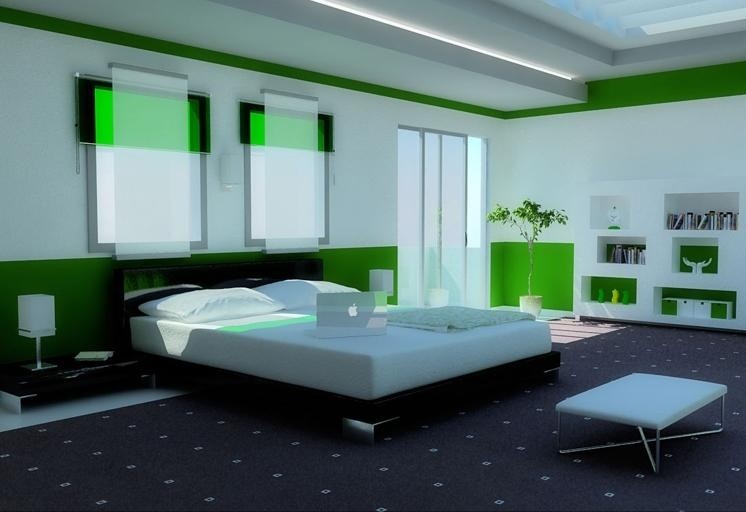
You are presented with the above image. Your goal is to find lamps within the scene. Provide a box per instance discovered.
[221,153,242,191]
[369,269,393,304]
[16,293,59,372]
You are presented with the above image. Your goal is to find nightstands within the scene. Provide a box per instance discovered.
[1,348,156,412]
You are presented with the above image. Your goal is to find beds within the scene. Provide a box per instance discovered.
[115,258,562,443]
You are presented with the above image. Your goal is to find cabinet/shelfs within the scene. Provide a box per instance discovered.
[572,179,746,332]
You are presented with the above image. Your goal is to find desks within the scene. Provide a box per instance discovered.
[556,371,728,477]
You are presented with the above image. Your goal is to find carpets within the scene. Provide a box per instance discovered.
[4,328,745,511]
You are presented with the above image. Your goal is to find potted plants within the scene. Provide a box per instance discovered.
[485,200,567,317]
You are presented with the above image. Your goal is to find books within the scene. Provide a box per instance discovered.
[667,211,738,230]
[74,351,113,361]
[611,244,646,264]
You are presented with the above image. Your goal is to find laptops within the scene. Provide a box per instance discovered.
[300,291,388,340]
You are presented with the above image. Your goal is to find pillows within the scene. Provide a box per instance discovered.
[254,278,362,311]
[140,287,284,318]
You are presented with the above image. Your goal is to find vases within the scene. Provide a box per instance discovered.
[427,286,450,309]
